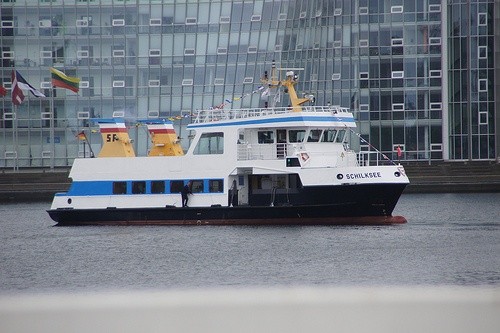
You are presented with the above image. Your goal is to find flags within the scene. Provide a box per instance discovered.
[16,71,46,99]
[11,70,25,106]
[50,66,80,94]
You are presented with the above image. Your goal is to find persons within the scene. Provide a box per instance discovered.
[181,182,193,207]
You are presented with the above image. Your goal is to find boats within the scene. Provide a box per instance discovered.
[45,60,411,226]
